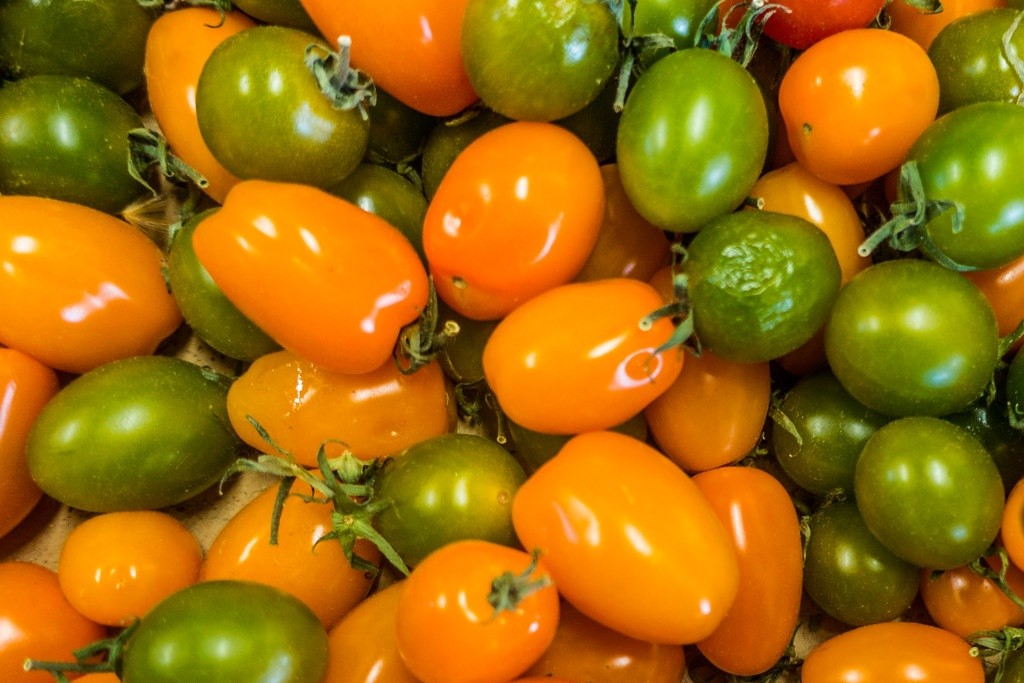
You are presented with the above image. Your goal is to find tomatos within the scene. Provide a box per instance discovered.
[0,0,1024,683]
